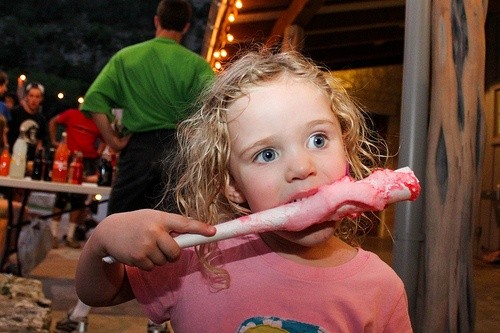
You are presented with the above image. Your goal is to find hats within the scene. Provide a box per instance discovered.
[27,82,44,94]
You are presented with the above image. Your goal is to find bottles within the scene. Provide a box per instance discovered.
[97,155,113,186]
[0,130,83,184]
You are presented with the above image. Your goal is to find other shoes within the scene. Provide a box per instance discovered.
[53,236,58,248]
[55,309,88,333]
[148,321,171,333]
[66,239,80,248]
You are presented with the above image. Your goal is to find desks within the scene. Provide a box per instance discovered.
[0,174,113,273]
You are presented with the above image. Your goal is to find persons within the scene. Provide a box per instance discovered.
[49,105,106,247]
[75,52,413,333]
[0,71,44,170]
[79,0,215,214]
